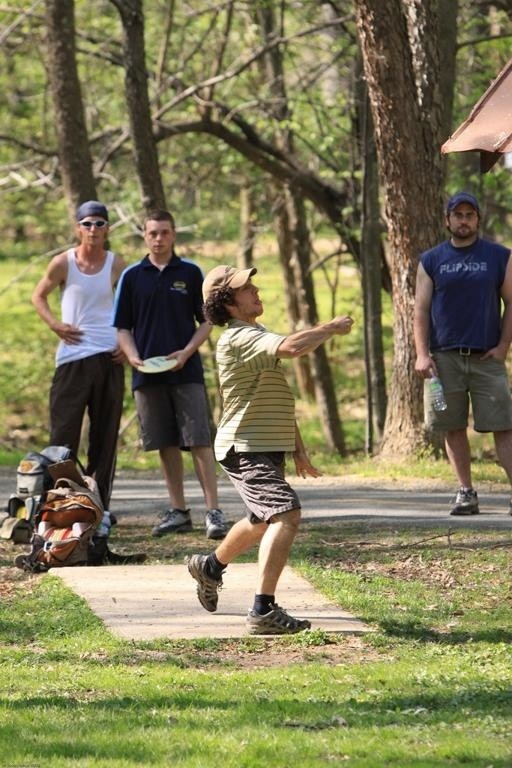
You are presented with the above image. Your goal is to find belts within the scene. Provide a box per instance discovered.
[432,345,493,357]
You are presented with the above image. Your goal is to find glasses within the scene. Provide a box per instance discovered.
[449,209,476,222]
[77,220,110,229]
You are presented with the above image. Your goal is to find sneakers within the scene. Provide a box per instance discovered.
[151,510,194,538]
[185,552,223,612]
[247,602,312,636]
[204,508,227,539]
[509,499,510,512]
[108,512,118,526]
[450,491,481,516]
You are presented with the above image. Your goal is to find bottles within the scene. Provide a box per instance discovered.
[429,369,448,412]
[92,510,111,560]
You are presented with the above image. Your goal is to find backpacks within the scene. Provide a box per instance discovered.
[0,445,93,546]
[13,462,146,576]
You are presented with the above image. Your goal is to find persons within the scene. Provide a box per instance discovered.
[111,210,230,541]
[413,193,512,517]
[31,202,130,526]
[188,262,355,636]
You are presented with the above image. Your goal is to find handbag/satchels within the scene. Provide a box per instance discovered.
[1,492,42,546]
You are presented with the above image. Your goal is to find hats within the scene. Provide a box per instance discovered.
[77,200,109,222]
[447,193,480,213]
[202,265,258,306]
